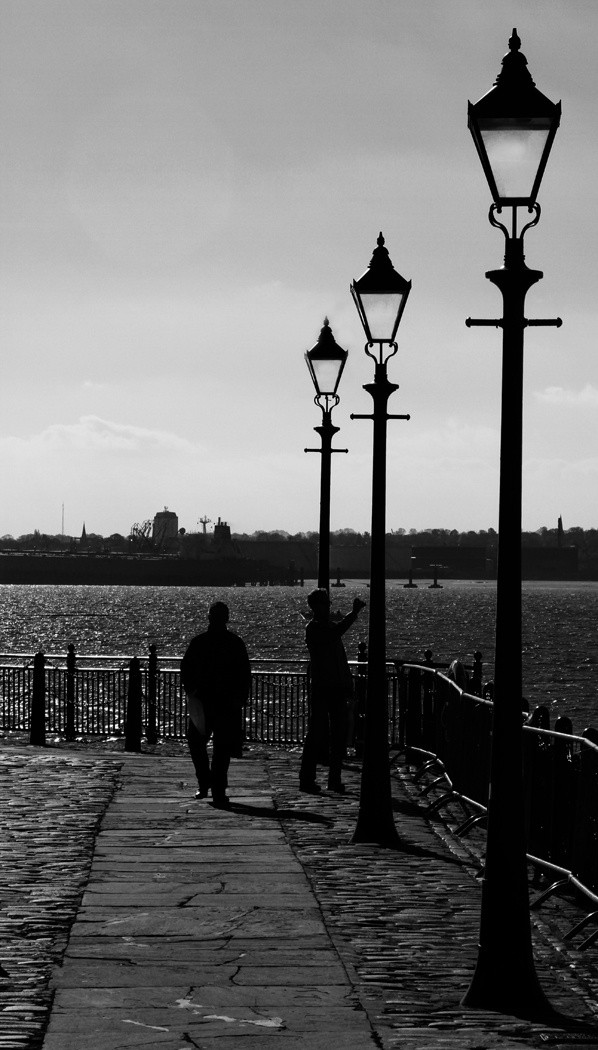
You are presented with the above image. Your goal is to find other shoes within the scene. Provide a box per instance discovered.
[195,781,213,799]
[213,795,230,808]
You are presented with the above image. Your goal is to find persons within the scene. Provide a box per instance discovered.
[299,589,365,795]
[180,602,253,805]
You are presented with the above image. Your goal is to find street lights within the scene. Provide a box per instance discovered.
[464,26,563,1020]
[303,314,352,589]
[349,231,415,848]
